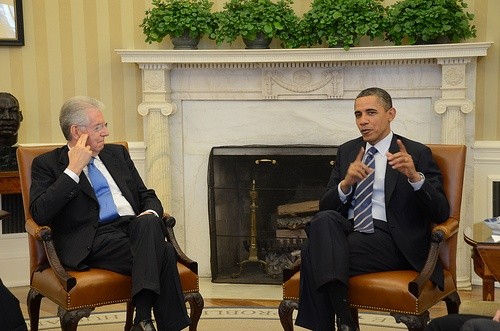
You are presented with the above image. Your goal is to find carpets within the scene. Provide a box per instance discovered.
[23,306,414,331]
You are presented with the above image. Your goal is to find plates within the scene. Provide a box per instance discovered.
[484,217,500,231]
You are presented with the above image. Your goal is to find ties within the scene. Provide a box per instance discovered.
[86,157,120,222]
[354,147,377,234]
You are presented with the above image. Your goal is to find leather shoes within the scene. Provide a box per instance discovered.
[131,319,155,331]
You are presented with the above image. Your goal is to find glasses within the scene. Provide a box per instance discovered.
[77,122,109,133]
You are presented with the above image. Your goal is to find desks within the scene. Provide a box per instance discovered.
[463,217,500,302]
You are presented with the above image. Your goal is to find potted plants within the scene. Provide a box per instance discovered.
[380,0,476,45]
[139,0,214,50]
[206,0,300,50]
[296,0,387,51]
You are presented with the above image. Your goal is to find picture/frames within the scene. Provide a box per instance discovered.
[0,0,26,46]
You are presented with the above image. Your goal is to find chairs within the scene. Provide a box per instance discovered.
[278,144,467,331]
[16,142,203,331]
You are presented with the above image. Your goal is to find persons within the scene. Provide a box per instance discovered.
[431,310,500,331]
[0,91,23,172]
[294,87,449,331]
[28,95,191,331]
